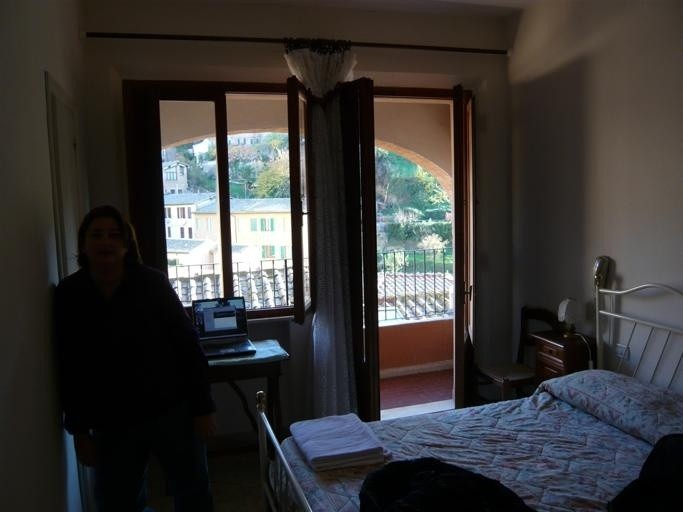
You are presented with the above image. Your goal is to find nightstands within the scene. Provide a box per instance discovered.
[529,328,596,388]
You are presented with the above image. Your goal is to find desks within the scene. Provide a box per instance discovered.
[207,339,290,457]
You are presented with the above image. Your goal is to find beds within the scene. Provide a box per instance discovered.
[255,273,683,512]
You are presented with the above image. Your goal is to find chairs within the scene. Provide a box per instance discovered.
[472,306,566,403]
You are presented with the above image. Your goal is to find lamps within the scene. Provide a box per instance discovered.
[558,297,585,339]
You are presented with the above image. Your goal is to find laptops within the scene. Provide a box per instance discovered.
[192,297,256,358]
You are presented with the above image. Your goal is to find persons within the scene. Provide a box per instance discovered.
[49,201,217,512]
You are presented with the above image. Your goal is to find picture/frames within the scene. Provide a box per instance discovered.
[45,73,82,292]
